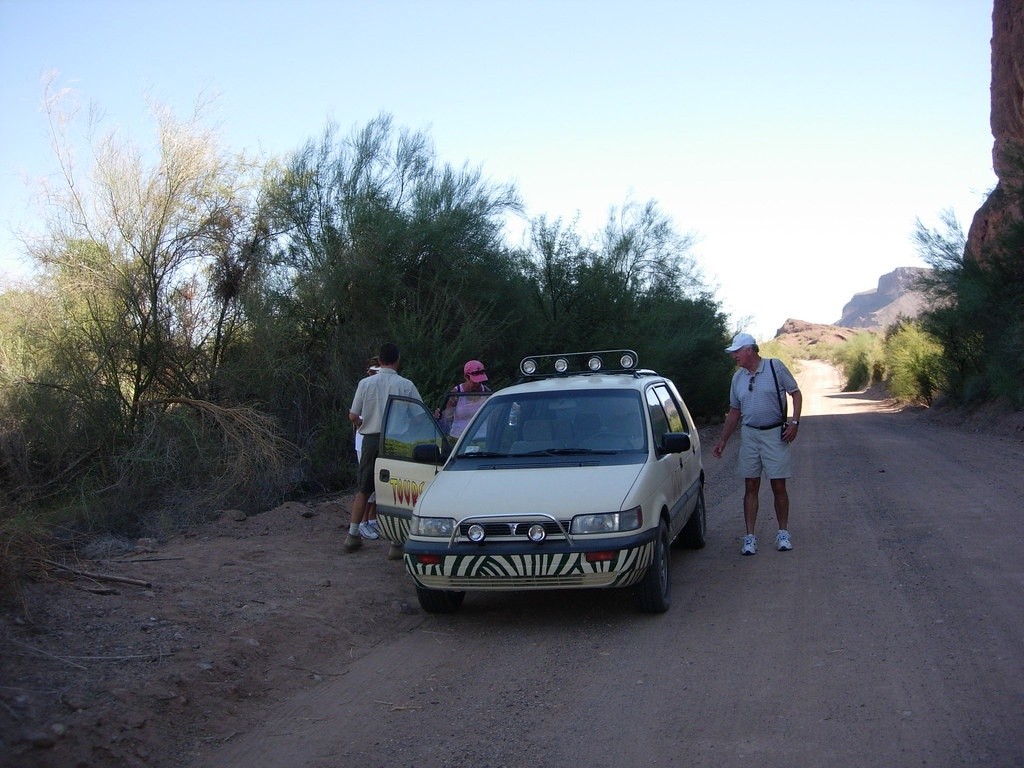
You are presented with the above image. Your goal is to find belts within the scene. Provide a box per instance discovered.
[744,422,785,430]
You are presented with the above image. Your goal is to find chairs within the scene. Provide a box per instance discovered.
[571,413,601,448]
[510,421,567,455]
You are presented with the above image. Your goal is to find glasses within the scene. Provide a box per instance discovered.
[748,377,755,392]
[466,369,486,376]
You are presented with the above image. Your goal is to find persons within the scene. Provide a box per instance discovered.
[712,333,802,555]
[433,360,519,451]
[342,343,428,559]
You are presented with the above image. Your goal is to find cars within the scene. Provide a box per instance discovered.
[372,349,705,617]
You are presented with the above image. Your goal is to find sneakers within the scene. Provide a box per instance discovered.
[740,534,759,555]
[358,521,379,539]
[774,529,792,552]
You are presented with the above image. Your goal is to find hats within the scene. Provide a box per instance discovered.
[464,360,488,383]
[725,332,755,353]
[367,357,381,373]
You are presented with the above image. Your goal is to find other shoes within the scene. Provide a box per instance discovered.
[344,532,364,550]
[387,545,402,560]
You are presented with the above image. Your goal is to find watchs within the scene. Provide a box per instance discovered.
[791,420,799,425]
